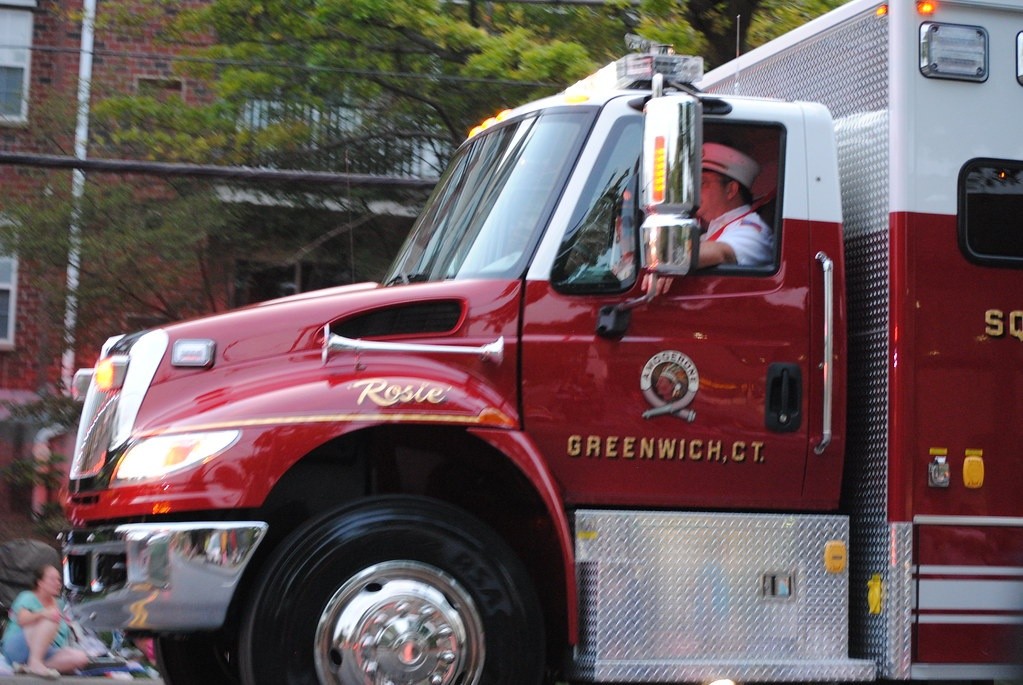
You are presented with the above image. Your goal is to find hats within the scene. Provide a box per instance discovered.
[701,141,764,189]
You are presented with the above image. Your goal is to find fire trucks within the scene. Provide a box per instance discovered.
[58,2,1023,683]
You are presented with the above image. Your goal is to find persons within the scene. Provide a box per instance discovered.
[3,564,88,678]
[596,143,773,296]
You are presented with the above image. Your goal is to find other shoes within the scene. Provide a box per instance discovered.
[23,663,62,680]
[12,661,30,675]
